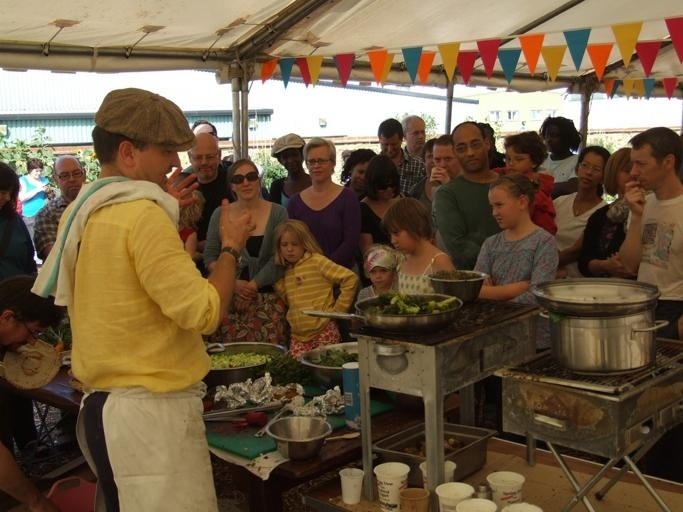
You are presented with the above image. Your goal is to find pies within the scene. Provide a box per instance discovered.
[4,340,60,389]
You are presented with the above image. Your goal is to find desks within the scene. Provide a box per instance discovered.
[31,350,477,510]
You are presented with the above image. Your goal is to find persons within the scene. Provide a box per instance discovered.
[34,88,260,511]
[1,115,683,511]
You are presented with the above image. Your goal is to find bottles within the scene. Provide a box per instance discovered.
[340,361,362,431]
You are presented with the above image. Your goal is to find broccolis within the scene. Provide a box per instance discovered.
[364,292,457,316]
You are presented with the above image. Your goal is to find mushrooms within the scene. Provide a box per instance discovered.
[211,370,345,419]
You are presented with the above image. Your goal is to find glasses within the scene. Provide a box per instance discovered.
[23,320,44,339]
[55,167,83,180]
[306,159,332,164]
[502,156,529,164]
[230,172,259,184]
[376,183,396,190]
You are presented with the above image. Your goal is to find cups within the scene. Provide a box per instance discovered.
[429,167,442,187]
[373,461,409,511]
[339,466,364,504]
[399,458,545,511]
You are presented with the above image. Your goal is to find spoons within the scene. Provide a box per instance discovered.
[324,432,361,442]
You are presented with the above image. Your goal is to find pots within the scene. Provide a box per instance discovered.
[532,307,670,376]
[298,290,463,335]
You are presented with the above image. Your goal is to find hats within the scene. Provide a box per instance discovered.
[270,133,305,157]
[95,88,197,152]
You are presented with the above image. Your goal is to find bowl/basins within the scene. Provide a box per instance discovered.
[430,267,488,304]
[203,339,287,385]
[529,276,665,317]
[263,416,332,463]
[294,341,358,387]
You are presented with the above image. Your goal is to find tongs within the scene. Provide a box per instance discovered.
[199,401,291,424]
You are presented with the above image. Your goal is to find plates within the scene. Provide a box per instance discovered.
[58,350,70,367]
